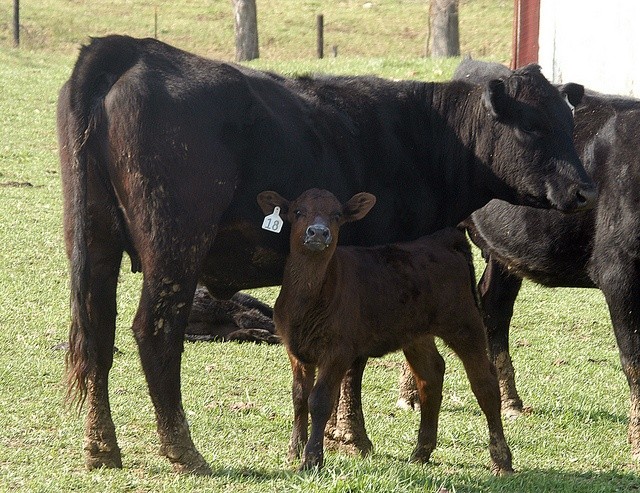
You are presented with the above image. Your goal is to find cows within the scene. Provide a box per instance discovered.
[183,286,280,343]
[54,30,600,477]
[395,50,640,453]
[254,186,515,478]
